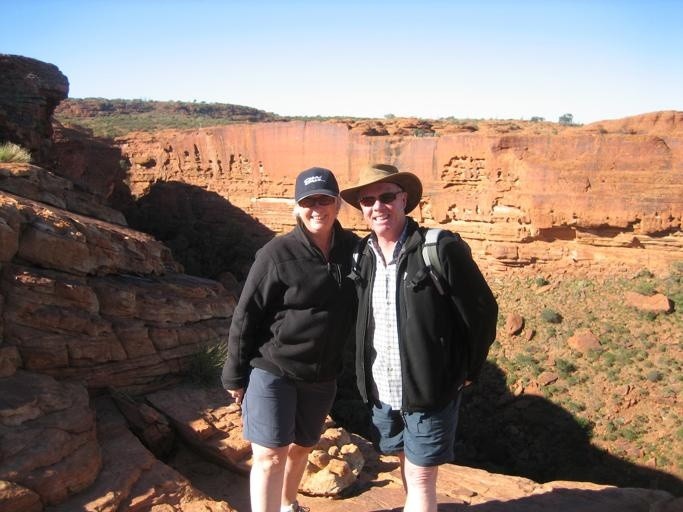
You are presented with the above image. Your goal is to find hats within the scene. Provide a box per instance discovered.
[340,163,423,214]
[294,167,339,204]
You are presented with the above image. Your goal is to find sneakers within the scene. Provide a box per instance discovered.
[290,501,309,512]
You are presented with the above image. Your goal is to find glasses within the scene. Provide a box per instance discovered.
[357,192,400,207]
[297,194,335,209]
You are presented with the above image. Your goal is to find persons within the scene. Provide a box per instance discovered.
[339,165,498,512]
[220,168,362,512]
